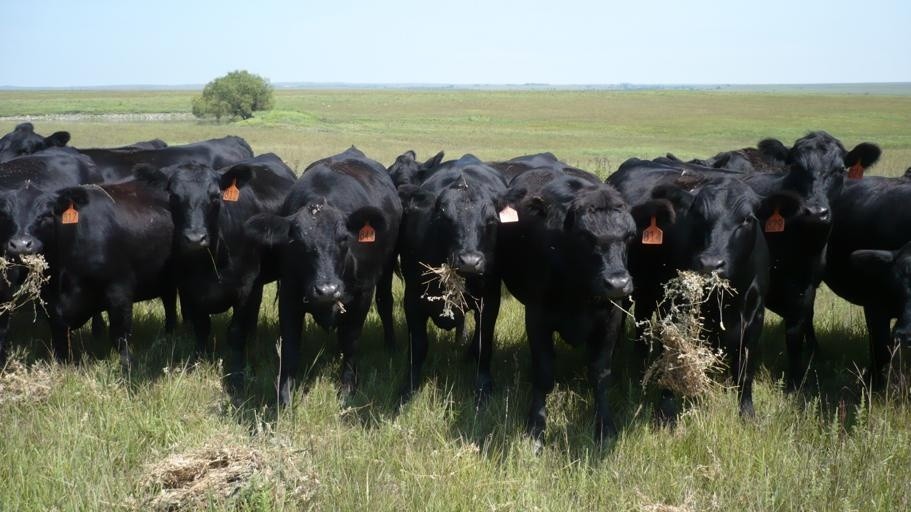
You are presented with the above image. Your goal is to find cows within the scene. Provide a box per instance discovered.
[0,122,911,456]
